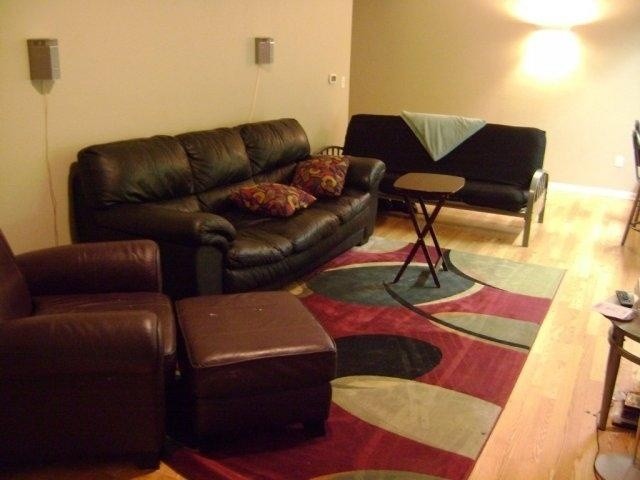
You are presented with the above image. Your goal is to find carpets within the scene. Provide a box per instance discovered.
[159,236,567,480]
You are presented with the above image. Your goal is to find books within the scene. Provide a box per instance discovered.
[612,391,640,432]
[592,299,632,320]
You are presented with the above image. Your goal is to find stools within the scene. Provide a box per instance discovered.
[174,289,338,454]
[598,279,640,431]
[393,172,466,288]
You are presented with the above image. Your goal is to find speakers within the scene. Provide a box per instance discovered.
[26,37,63,82]
[254,35,277,65]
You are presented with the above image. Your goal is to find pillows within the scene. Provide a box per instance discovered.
[289,155,353,198]
[227,182,318,217]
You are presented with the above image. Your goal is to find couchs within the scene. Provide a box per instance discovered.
[69,117,387,296]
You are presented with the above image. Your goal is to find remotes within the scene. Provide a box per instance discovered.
[616,290,633,308]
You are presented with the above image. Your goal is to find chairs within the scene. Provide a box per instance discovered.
[0,227,177,470]
[620,119,640,247]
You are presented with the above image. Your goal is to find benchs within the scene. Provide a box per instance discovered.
[342,114,549,246]
